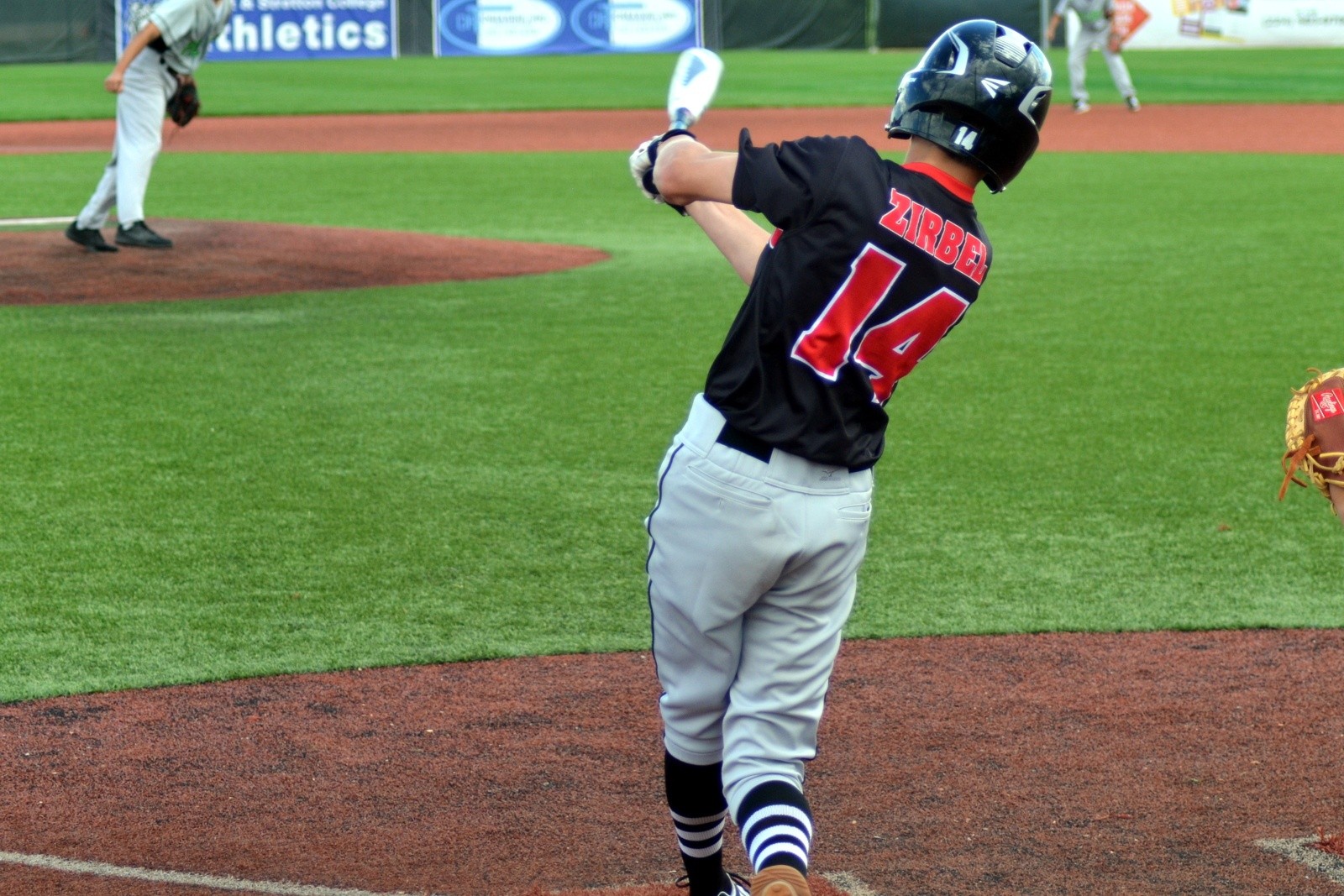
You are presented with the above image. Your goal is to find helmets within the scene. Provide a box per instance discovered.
[884,17,1054,196]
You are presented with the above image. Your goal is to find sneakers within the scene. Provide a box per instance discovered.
[1126,95,1143,112]
[1072,98,1090,115]
[749,864,811,896]
[114,220,173,250]
[675,870,751,896]
[65,220,119,253]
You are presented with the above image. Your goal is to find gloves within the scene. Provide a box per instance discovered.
[628,128,697,217]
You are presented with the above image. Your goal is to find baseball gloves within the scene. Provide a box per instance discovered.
[1285,368,1344,522]
[166,83,199,126]
[1108,33,1121,52]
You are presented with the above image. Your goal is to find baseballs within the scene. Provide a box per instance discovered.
[642,47,725,196]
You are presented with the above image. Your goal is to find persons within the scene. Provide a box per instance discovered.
[65,0,235,251]
[1047,0,1141,115]
[630,18,1052,896]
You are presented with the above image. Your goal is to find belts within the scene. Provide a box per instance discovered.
[716,423,871,474]
[159,58,179,78]
